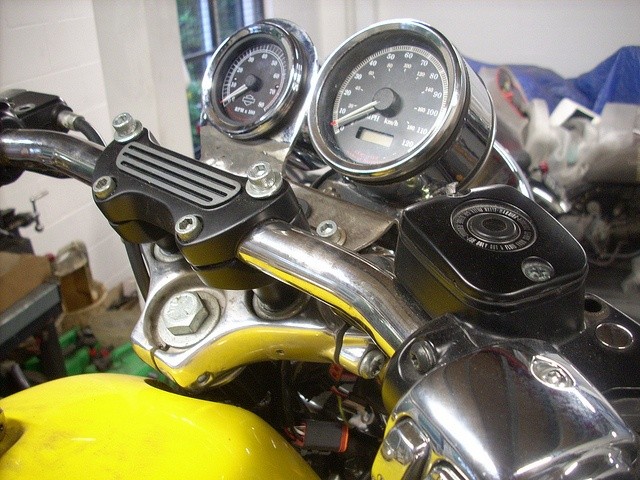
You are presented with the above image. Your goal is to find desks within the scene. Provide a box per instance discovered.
[0,245,68,378]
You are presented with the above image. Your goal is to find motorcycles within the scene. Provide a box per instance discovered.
[1,17,640,478]
